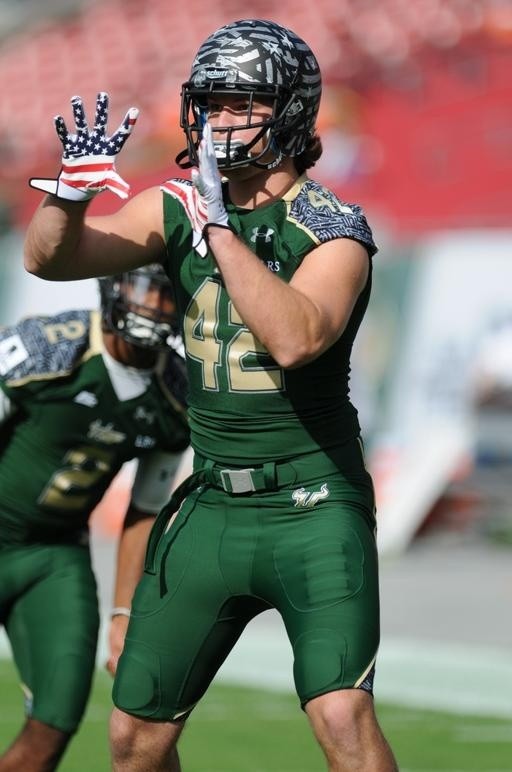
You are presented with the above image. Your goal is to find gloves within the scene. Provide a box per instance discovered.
[158,120,238,259]
[28,88,140,205]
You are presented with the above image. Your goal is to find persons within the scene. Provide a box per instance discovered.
[0,261,190,772]
[24,20,398,770]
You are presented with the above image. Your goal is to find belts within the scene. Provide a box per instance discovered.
[142,433,366,575]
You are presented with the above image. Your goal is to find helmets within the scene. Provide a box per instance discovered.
[96,261,182,354]
[176,18,324,172]
